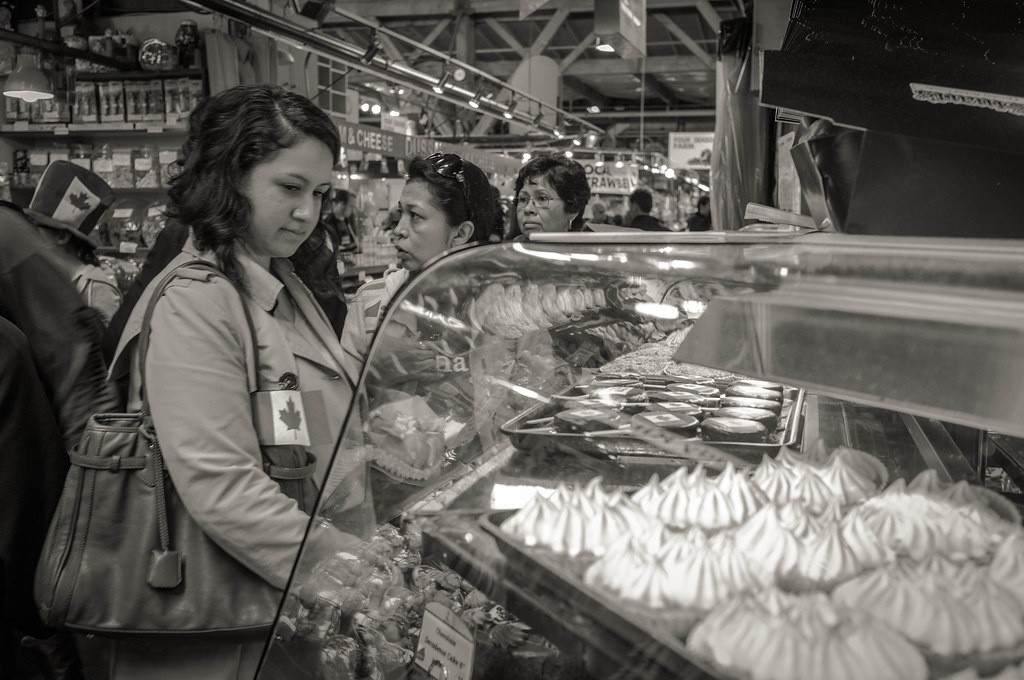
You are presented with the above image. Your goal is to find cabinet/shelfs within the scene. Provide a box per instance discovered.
[11,41,210,255]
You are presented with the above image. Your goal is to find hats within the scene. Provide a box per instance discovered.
[23,160,116,250]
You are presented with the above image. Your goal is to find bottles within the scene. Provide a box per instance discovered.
[176,20,200,50]
[12,142,186,249]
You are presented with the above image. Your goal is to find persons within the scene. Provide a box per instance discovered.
[91,85,395,678]
[0,155,714,680]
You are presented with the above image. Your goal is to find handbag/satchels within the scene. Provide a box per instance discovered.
[32,260,321,637]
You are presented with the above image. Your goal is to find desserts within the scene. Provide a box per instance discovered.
[500,444,1024,680]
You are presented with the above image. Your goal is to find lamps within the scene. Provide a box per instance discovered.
[2,51,55,103]
[433,63,455,94]
[469,76,489,109]
[593,154,604,167]
[614,153,624,168]
[530,103,545,128]
[553,114,567,136]
[573,125,587,146]
[503,91,518,119]
[360,30,385,67]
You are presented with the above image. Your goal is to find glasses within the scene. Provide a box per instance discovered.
[423,151,475,218]
[511,194,561,209]
